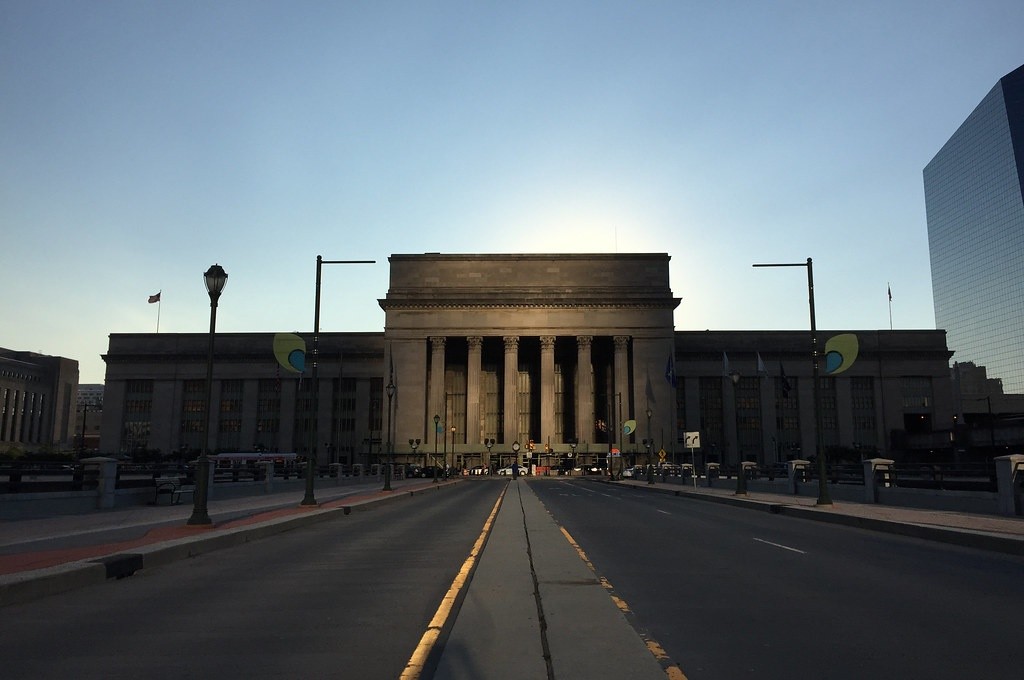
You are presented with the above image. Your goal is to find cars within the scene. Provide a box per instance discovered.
[497,465,529,476]
[565,464,599,475]
[622,464,645,478]
[410,465,442,478]
[469,465,489,475]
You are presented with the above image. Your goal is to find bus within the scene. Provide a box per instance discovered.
[195,452,299,479]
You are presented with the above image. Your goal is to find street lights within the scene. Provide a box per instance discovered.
[187,262,229,524]
[382,378,396,492]
[451,425,456,467]
[545,444,548,475]
[433,415,441,483]
[79,403,103,451]
[611,392,623,465]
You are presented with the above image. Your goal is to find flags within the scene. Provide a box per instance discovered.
[719,355,735,392]
[148,293,160,303]
[664,355,676,387]
[782,368,792,398]
[888,286,892,301]
[757,353,768,376]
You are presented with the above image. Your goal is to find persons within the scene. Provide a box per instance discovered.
[463,467,469,475]
[509,461,522,480]
[482,463,486,474]
[493,463,498,473]
